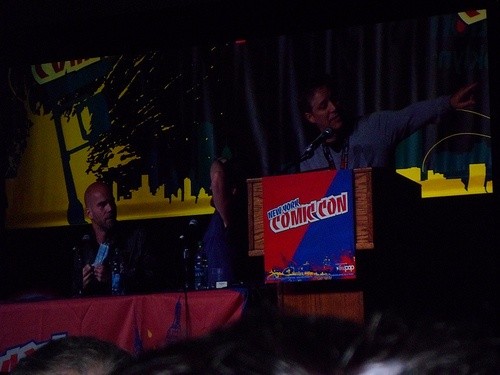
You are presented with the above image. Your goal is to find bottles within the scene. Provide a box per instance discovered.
[110,248,127,296]
[193,240,209,290]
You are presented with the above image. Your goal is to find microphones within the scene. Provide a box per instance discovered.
[300,127,333,157]
[73,234,90,252]
[180,220,197,241]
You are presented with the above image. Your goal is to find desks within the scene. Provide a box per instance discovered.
[0,285,275,374]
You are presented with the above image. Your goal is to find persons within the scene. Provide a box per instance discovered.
[9,307,500,375]
[294,72,478,227]
[59,181,174,298]
[194,158,264,301]
